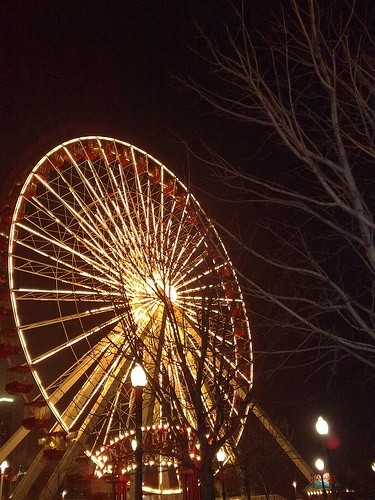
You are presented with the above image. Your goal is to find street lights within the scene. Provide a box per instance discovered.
[315,457,328,500]
[316,416,339,500]
[0,278,299,500]
